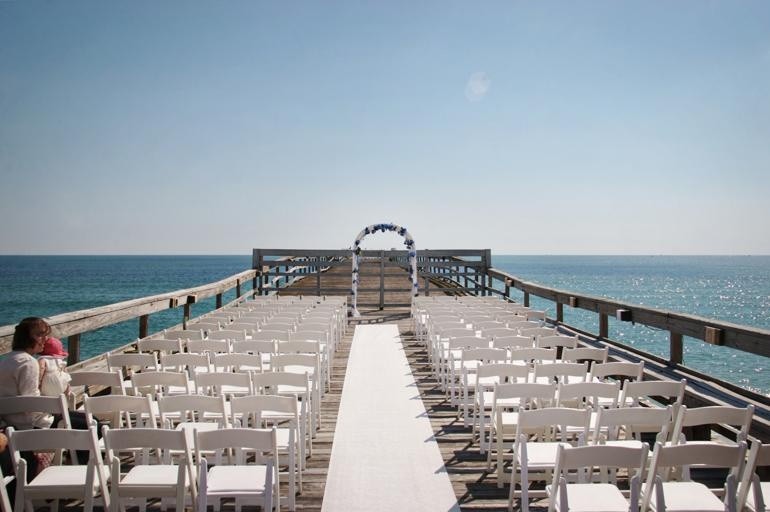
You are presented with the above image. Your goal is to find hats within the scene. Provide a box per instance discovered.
[37,337,69,356]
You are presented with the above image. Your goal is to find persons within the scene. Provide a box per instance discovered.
[37,337,73,398]
[0,316,103,465]
